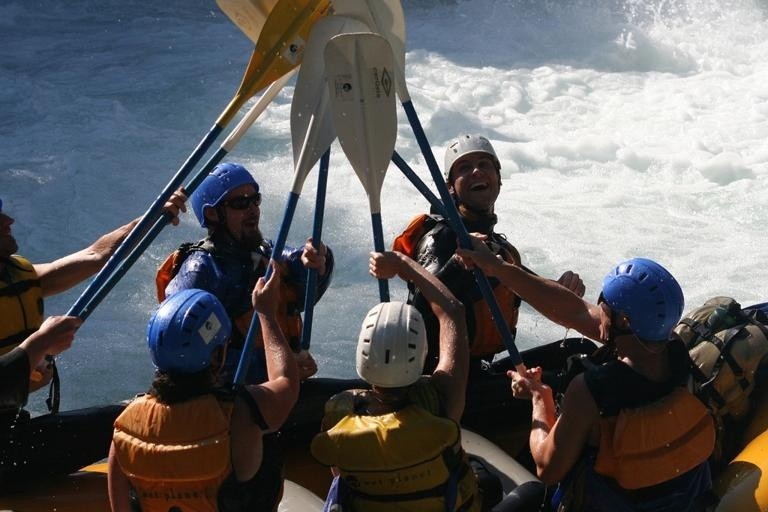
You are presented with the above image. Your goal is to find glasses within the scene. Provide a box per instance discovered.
[215,192,262,210]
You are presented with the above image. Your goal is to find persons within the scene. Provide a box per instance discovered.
[0,184,189,498]
[156,162,335,512]
[106,257,300,512]
[310,250,470,512]
[1,313,83,411]
[389,133,586,377]
[456,233,721,512]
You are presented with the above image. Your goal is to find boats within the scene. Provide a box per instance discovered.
[0,294,764,511]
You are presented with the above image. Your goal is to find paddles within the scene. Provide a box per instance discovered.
[228,15,372,391]
[332,0,528,382]
[321,30,398,300]
[215,1,541,279]
[40,1,332,366]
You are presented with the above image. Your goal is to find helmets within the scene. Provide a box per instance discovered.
[600,256,685,344]
[145,287,233,381]
[442,133,501,186]
[189,161,260,228]
[353,302,430,390]
